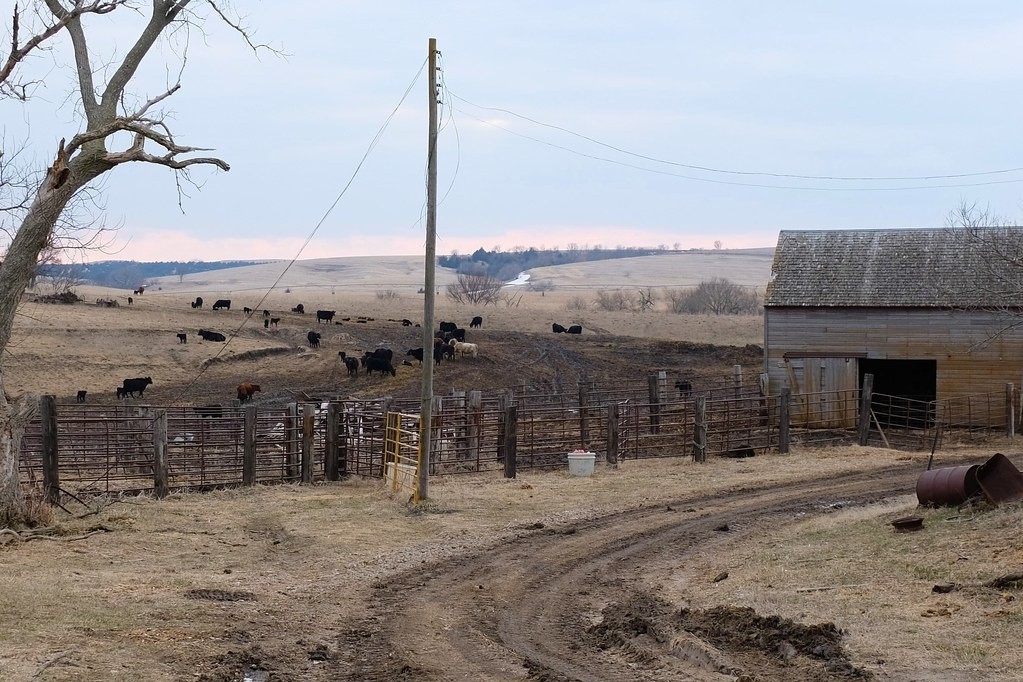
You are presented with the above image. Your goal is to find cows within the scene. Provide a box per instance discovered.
[263,310,270,317]
[317,310,336,323]
[197,329,226,343]
[127,287,144,304]
[116,376,153,399]
[270,317,280,326]
[77,390,87,402]
[552,323,582,334]
[470,316,482,328]
[674,381,693,398]
[338,348,396,378]
[237,383,262,403]
[244,307,252,313]
[191,297,231,310]
[193,403,223,424]
[402,319,479,366]
[308,331,321,348]
[292,304,304,314]
[264,319,269,328]
[176,334,187,343]
[334,317,375,325]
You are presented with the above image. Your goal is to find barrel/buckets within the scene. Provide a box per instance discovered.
[916,465,981,509]
[567,452,596,476]
